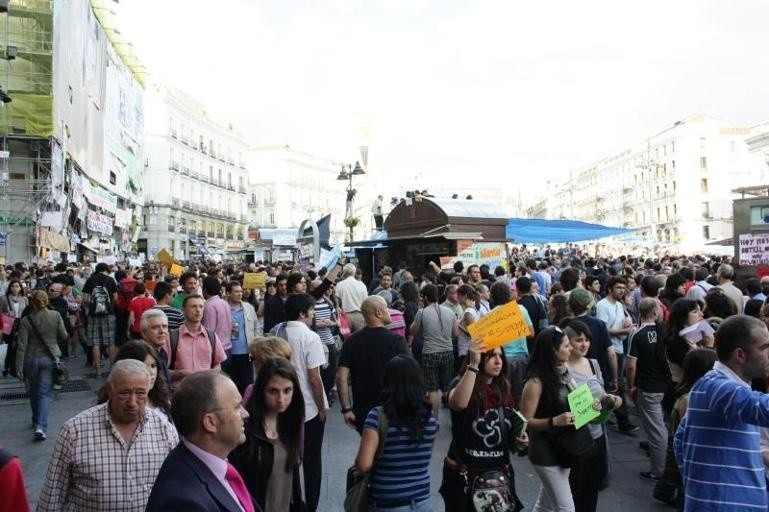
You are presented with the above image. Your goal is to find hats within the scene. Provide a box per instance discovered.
[47,283,64,299]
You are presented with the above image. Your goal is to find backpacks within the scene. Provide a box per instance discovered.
[90,274,113,317]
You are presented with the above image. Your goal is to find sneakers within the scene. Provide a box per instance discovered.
[639,440,650,450]
[34,430,48,441]
[605,420,616,426]
[639,470,660,481]
[617,420,641,434]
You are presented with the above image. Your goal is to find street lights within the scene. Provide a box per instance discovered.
[336,159,365,245]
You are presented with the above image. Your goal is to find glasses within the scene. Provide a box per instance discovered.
[610,286,626,289]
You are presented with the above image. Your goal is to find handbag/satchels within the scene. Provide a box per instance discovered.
[471,469,516,511]
[0,343,9,373]
[1,312,15,336]
[55,364,68,386]
[343,465,373,512]
[653,482,685,508]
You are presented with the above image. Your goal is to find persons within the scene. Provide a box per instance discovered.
[387,190,474,216]
[370,195,385,232]
[345,183,359,218]
[1,244,769,511]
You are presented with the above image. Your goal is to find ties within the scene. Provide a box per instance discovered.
[225,462,257,512]
